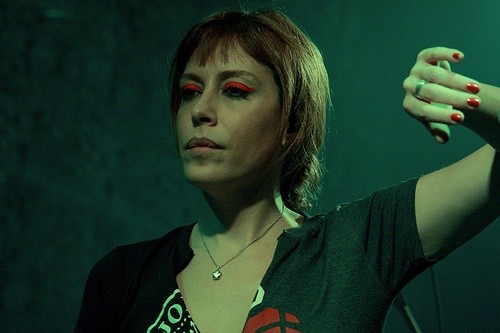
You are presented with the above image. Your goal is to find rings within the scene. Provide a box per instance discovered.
[415,78,429,96]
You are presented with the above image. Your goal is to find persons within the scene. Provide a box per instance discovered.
[74,5,500,333]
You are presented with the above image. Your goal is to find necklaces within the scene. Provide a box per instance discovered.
[194,198,286,281]
